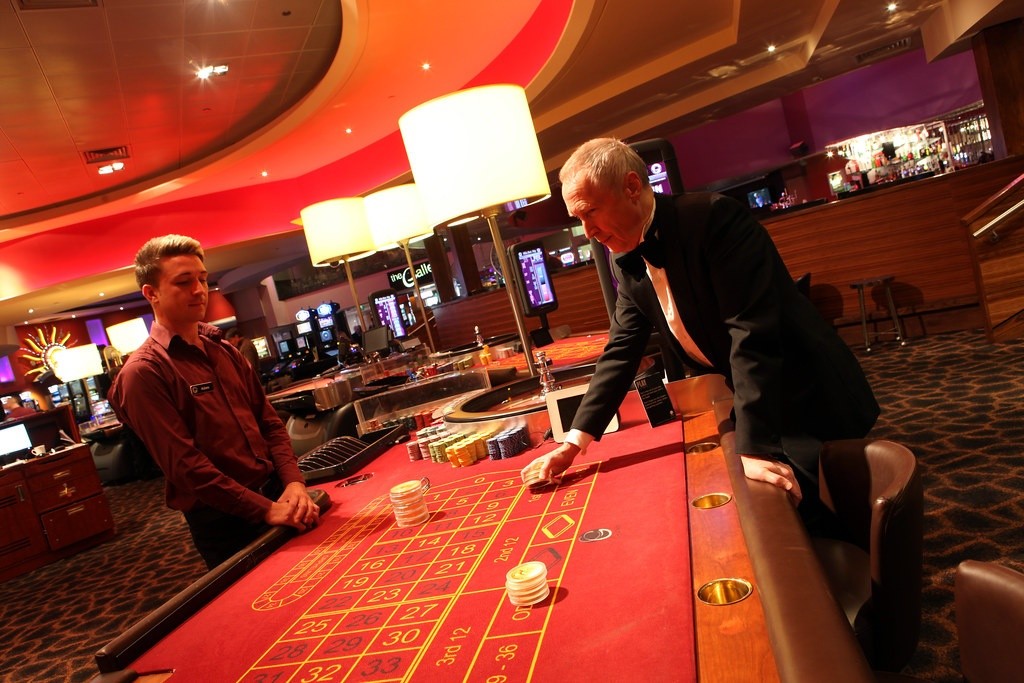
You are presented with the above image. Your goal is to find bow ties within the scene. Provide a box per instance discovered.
[614,218,678,283]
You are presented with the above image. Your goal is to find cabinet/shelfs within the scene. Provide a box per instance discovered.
[0,404,115,584]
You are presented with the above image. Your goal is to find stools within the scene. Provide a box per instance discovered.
[850,276,907,353]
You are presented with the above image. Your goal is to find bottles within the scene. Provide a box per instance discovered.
[861,141,949,185]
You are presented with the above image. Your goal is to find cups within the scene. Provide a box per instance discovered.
[31,445,46,456]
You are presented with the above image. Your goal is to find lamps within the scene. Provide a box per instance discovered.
[366,183,436,353]
[300,196,375,333]
[398,83,551,375]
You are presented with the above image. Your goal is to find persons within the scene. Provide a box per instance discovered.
[335,329,352,363]
[752,192,768,208]
[517,135,880,606]
[350,325,364,348]
[105,232,321,573]
[224,326,263,386]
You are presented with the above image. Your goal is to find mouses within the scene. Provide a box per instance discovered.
[54,446,65,452]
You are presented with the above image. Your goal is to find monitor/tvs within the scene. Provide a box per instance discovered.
[367,289,409,341]
[0,420,34,459]
[507,239,559,318]
[627,138,686,196]
